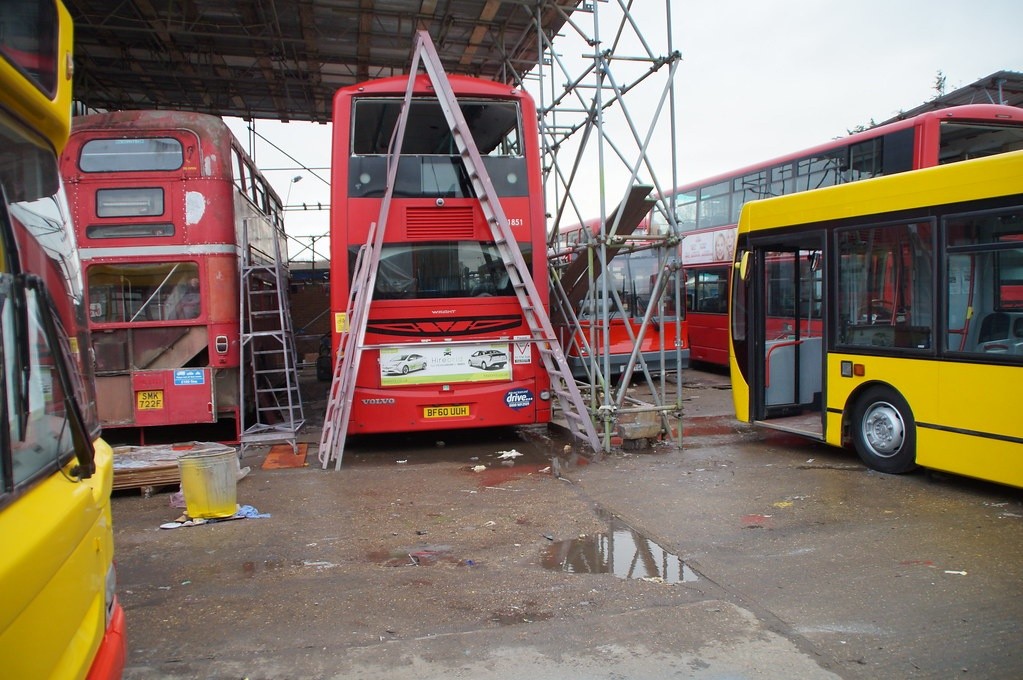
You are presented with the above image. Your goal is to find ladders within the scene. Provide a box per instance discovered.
[314,24,607,474]
[237,213,307,459]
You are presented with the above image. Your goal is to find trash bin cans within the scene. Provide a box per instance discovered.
[176,445,241,518]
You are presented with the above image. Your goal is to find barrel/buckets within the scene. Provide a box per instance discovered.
[177,447,238,519]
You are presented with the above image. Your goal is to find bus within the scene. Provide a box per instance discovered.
[648,103,1023,366]
[0,0,128,680]
[58,111,285,447]
[548,215,690,378]
[729,149,1023,488]
[331,73,553,434]
